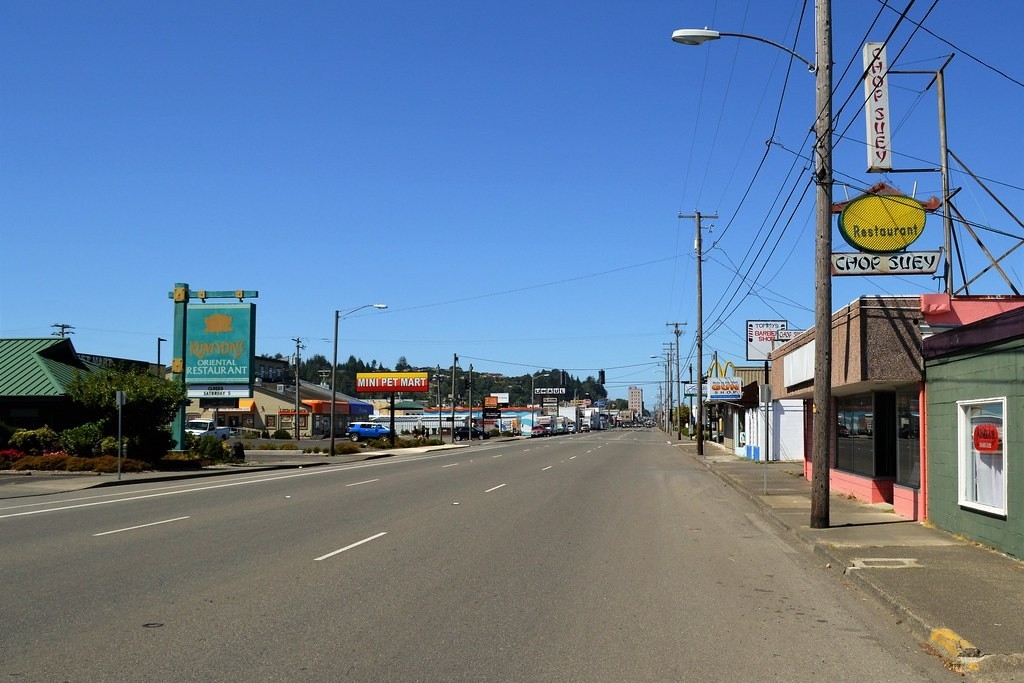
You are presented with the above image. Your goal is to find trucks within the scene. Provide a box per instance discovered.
[537,415,558,437]
[555,417,569,434]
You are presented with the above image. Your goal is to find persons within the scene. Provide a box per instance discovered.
[411,425,430,440]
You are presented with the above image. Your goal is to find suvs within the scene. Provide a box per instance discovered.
[453,426,490,441]
[345,422,397,442]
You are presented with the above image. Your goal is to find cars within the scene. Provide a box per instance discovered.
[530,424,548,438]
[581,424,590,433]
[568,424,577,433]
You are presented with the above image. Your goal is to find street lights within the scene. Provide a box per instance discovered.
[156,337,168,380]
[653,370,668,433]
[649,354,682,440]
[671,28,830,529]
[329,303,389,457]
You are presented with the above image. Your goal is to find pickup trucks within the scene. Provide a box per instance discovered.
[184,418,230,443]
